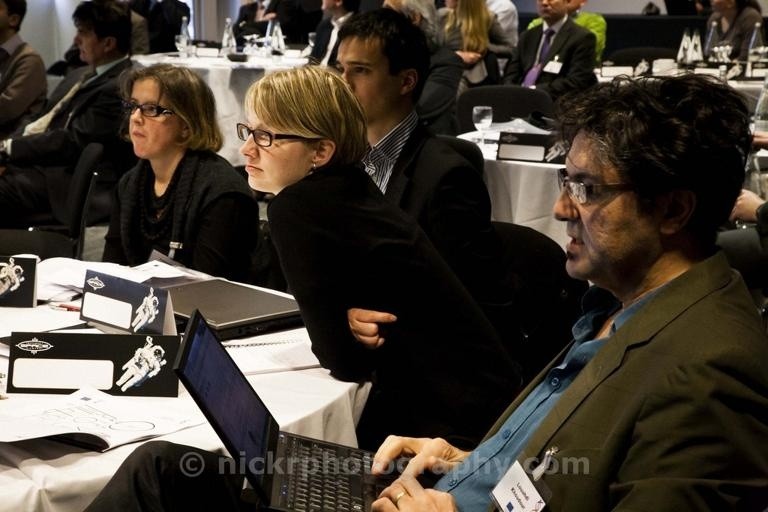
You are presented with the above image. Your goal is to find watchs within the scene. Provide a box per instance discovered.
[0,140,9,160]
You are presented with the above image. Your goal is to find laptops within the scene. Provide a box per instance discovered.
[153,276,303,342]
[171,308,426,512]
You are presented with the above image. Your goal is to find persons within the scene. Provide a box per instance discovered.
[545,139,570,163]
[130,0,190,53]
[232,0,296,47]
[0,0,148,230]
[44,0,150,77]
[717,186,768,279]
[132,288,160,333]
[503,0,600,120]
[380,0,465,137]
[311,0,359,66]
[443,0,517,98]
[236,65,532,453]
[528,0,607,66]
[707,0,764,61]
[0,258,26,296]
[116,336,168,392]
[84,74,768,512]
[486,0,520,76]
[0,0,48,141]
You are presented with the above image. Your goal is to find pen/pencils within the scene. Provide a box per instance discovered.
[58,304,81,311]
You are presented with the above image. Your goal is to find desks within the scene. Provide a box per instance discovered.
[455,119,573,251]
[1,257,364,511]
[135,43,309,167]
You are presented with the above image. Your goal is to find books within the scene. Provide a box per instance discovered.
[220,327,323,377]
[0,386,209,454]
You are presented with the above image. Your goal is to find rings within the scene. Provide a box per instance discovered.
[394,491,408,504]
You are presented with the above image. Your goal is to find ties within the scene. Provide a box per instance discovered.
[27,68,97,135]
[254,4,265,23]
[522,28,556,88]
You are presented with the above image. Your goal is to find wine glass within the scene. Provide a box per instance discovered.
[473,106,492,151]
[175,35,188,62]
[308,33,316,47]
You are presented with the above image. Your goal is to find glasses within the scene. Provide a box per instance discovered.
[236,121,322,148]
[123,98,179,119]
[552,167,637,208]
